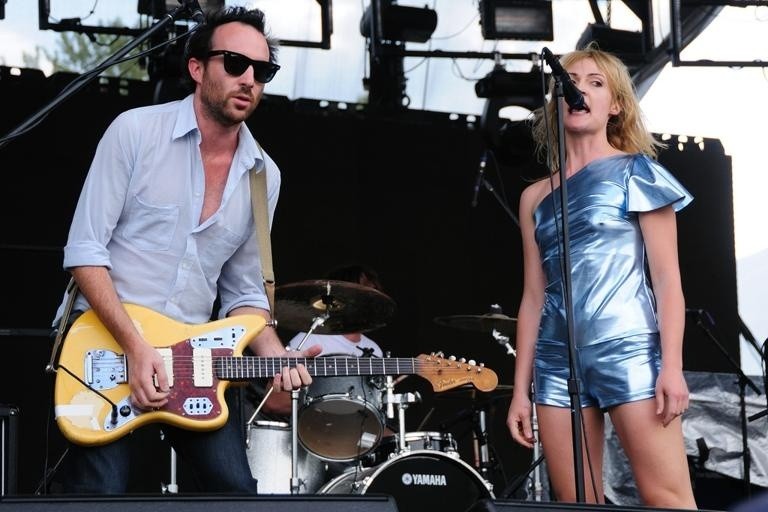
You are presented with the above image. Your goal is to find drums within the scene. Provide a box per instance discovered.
[298,352,385,462]
[315,448,495,507]
[243,421,327,495]
[374,432,460,462]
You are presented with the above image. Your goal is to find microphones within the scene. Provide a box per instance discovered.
[544,47,583,110]
[686,308,704,316]
[177,0,205,24]
[471,152,488,207]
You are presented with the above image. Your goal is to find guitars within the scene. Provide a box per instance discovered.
[53,302,498,448]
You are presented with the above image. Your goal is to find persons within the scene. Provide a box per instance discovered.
[504,33,703,511]
[51,7,312,500]
[259,257,400,444]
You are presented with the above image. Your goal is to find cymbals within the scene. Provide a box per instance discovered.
[433,384,516,401]
[447,314,520,335]
[274,281,395,335]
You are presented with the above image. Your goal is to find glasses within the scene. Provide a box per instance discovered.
[206,49,281,85]
